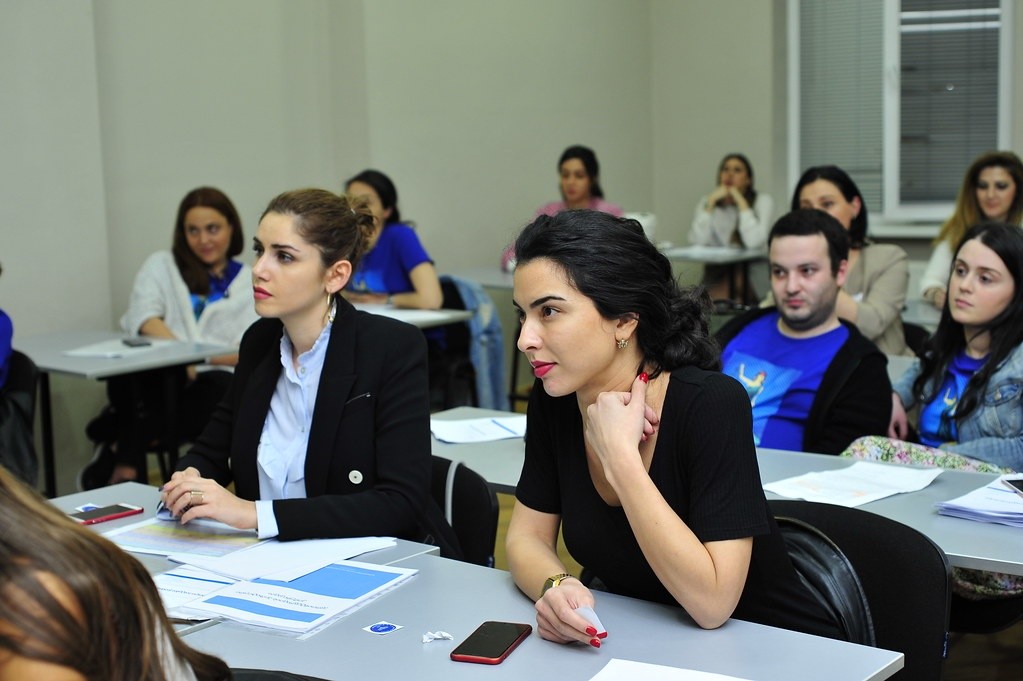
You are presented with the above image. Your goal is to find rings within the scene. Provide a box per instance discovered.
[190,491,204,504]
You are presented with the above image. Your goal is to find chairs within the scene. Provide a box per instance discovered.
[0,274,955,681]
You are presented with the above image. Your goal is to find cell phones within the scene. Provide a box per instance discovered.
[450,621,532,665]
[1002,479,1023,496]
[69,502,143,524]
[123,337,149,346]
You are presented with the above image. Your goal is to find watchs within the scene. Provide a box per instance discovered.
[540,573,573,597]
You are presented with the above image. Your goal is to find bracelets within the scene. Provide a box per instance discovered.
[387,294,392,304]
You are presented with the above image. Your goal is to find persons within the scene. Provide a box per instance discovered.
[687,154,775,302]
[839,220,1023,602]
[505,208,843,648]
[158,189,464,561]
[791,165,919,430]
[0,464,234,681]
[345,170,446,393]
[76,187,263,491]
[501,145,624,271]
[713,208,894,456]
[920,150,1023,310]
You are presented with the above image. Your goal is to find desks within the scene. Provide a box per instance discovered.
[181,555,905,681]
[663,245,766,306]
[18,329,239,499]
[353,300,475,328]
[902,298,944,325]
[431,406,1023,576]
[46,479,439,638]
[480,273,528,412]
